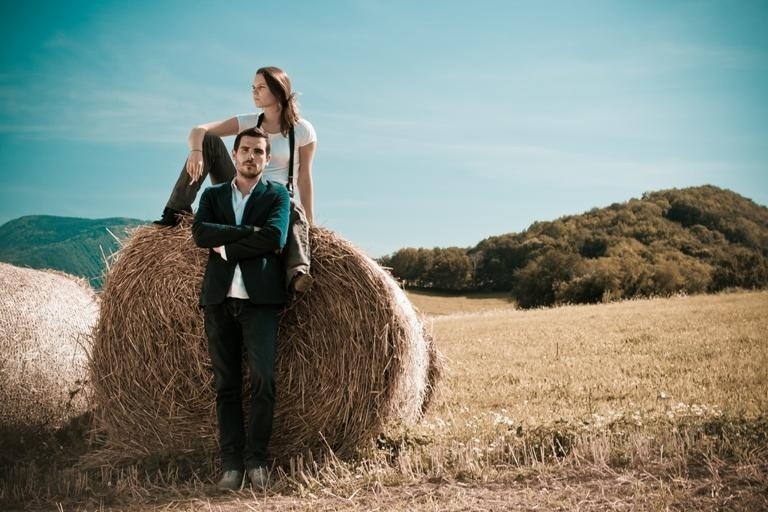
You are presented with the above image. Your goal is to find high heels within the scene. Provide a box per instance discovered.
[154,209,192,225]
[290,273,313,301]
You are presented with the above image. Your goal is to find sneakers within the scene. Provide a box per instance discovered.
[218,469,243,491]
[247,467,268,485]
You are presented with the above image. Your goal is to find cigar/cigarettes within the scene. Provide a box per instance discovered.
[189,178,195,186]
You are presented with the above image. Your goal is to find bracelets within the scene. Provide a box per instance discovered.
[190,148,202,152]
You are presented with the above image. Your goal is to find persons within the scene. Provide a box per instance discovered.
[191,127,290,492]
[153,66,316,292]
